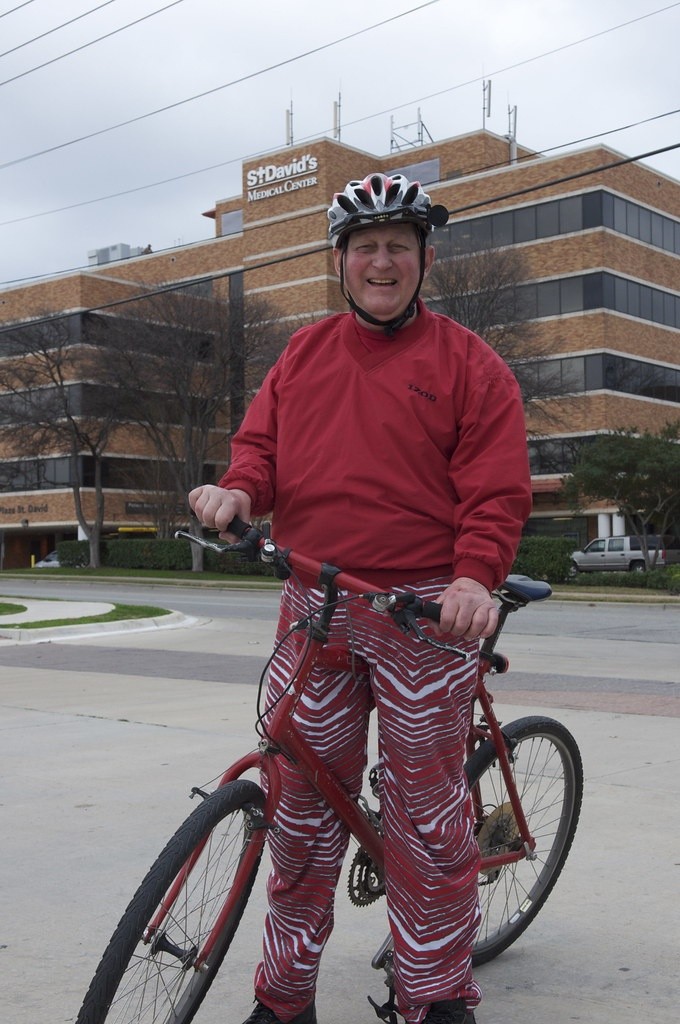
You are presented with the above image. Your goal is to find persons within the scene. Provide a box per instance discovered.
[187,171,532,1023]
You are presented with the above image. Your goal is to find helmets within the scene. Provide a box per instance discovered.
[325,174,435,241]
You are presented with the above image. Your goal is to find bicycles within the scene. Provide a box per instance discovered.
[73,502,585,1024]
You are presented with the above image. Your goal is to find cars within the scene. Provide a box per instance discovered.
[33,548,88,568]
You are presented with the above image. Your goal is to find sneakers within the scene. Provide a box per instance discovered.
[239,1000,318,1024]
[408,998,478,1022]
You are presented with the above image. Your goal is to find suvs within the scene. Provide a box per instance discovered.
[567,536,680,576]
